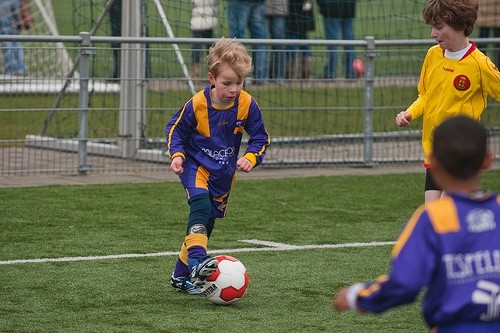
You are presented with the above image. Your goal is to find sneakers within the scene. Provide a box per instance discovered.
[171,271,202,295]
[190,256,218,288]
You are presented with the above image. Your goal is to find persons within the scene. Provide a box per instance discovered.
[394,0,499,206]
[473,0,500,70]
[335,114,500,333]
[107,0,153,80]
[190,0,358,80]
[165,37,271,295]
[0,1,30,78]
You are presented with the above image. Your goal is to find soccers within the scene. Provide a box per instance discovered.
[203,255,249,305]
